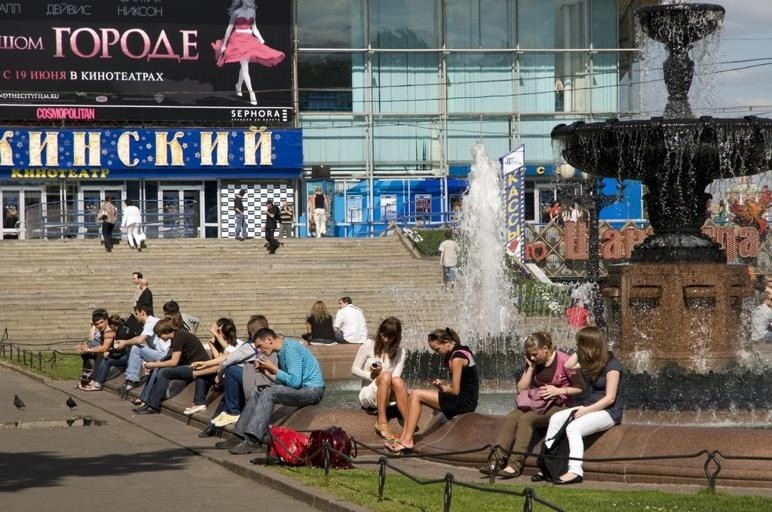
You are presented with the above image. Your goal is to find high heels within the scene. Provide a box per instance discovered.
[374,417,415,456]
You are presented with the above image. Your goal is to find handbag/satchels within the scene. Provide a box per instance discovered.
[310,224,316,232]
[517,387,568,413]
[138,233,146,242]
[98,210,107,220]
[269,426,311,464]
[537,429,570,483]
[309,427,351,469]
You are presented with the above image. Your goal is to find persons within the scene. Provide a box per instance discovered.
[265,200,281,254]
[351,316,419,440]
[479,331,583,478]
[313,185,330,238]
[278,201,293,240]
[333,297,369,344]
[705,201,727,219]
[211,0,286,105]
[120,199,142,250]
[750,291,772,343]
[531,326,627,484]
[215,328,326,454]
[301,301,336,344]
[383,328,479,455]
[566,298,591,331]
[234,189,251,240]
[101,196,118,252]
[548,201,584,224]
[71,272,278,428]
[438,231,461,289]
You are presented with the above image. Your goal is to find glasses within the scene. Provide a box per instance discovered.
[429,334,450,340]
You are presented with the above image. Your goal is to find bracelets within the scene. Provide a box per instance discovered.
[563,388,567,396]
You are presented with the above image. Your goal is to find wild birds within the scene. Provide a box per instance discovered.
[66,396,77,410]
[14,394,26,410]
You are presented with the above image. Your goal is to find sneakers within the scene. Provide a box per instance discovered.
[119,380,142,393]
[184,405,206,416]
[129,398,160,414]
[210,412,240,427]
[230,437,262,454]
[235,235,243,241]
[74,370,103,391]
[216,436,244,449]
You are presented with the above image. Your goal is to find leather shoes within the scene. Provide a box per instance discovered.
[554,474,583,484]
[532,473,544,481]
[480,466,519,477]
